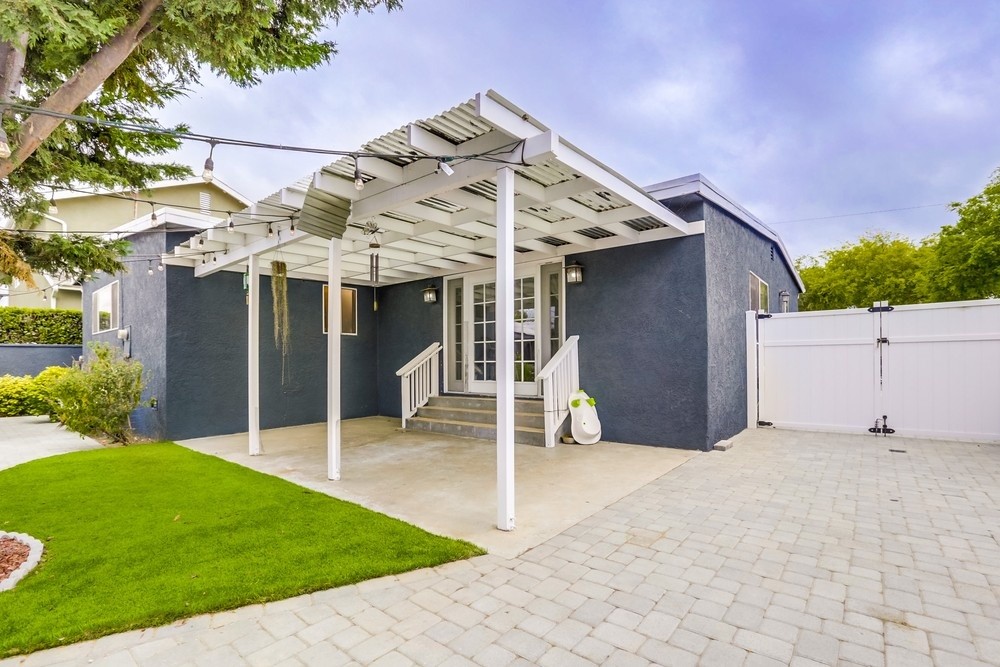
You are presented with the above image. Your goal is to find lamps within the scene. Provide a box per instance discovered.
[563,259,587,285]
[420,282,439,305]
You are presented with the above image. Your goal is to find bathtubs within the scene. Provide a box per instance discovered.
[568,389,602,445]
